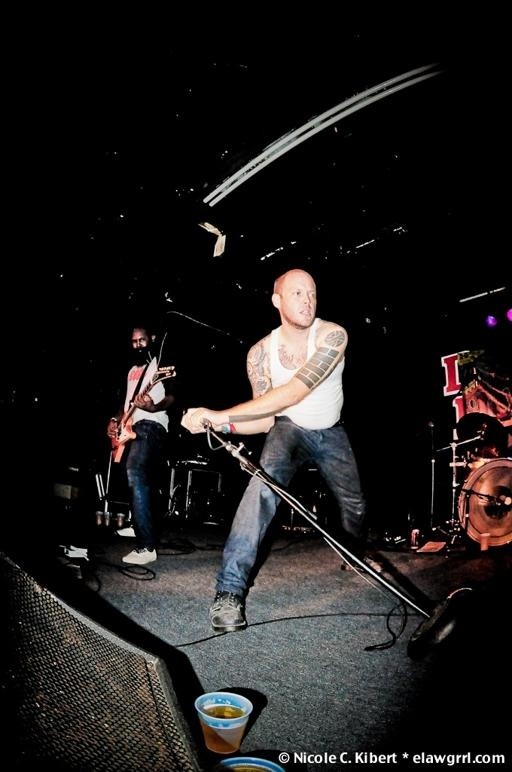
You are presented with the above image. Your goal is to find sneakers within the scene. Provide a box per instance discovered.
[121,548,159,565]
[338,553,386,576]
[117,527,137,539]
[206,589,249,632]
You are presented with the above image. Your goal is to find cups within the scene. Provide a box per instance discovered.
[195,691,254,755]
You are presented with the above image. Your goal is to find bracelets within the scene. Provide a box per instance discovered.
[110,417,117,421]
[222,424,236,435]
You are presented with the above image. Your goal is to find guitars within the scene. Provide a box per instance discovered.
[112,366,177,463]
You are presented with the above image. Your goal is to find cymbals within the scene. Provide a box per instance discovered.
[454,456,511,546]
[456,412,508,456]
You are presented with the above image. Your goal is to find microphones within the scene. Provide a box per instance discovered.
[183,410,215,432]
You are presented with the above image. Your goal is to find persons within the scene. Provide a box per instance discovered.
[179,268,383,633]
[105,322,176,566]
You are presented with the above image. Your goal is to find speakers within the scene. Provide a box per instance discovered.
[0,554,205,772]
